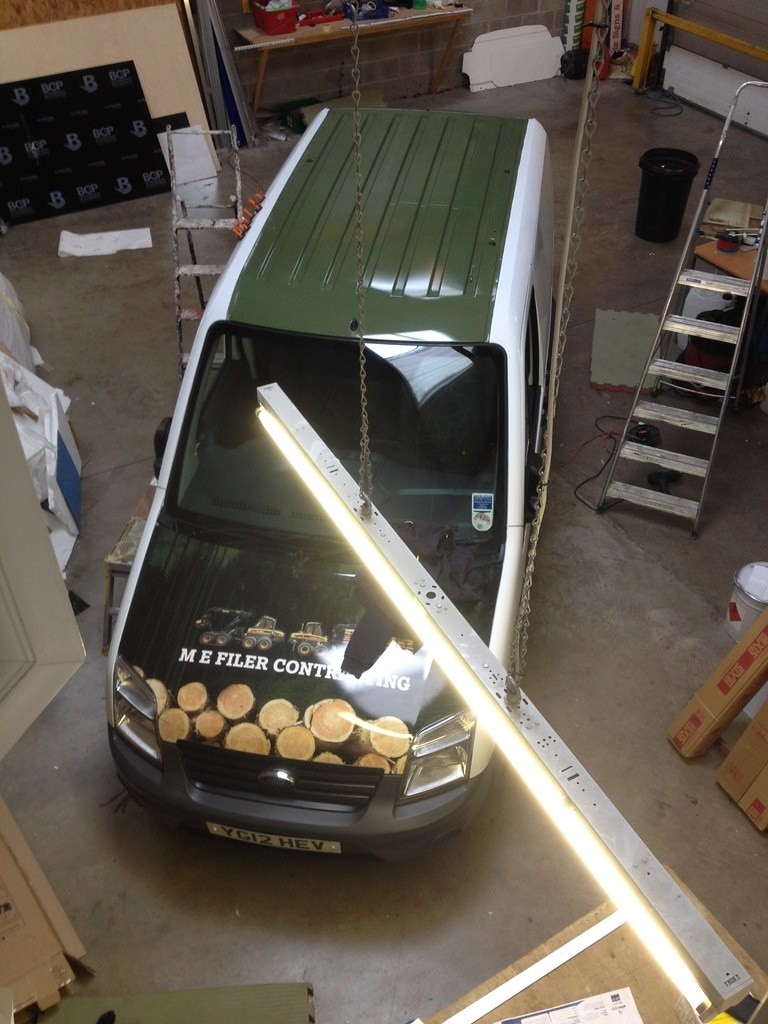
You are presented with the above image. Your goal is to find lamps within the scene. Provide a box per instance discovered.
[255,381,754,1024]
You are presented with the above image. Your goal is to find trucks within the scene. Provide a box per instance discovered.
[101,107,560,864]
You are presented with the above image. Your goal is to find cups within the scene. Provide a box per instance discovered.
[454,0,463,9]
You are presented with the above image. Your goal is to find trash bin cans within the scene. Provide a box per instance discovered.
[635,147,700,243]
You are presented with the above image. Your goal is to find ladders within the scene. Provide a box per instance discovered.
[167,123,242,382]
[596,81,768,536]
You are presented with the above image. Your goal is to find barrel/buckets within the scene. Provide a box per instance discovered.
[724,561,768,644]
[635,147,697,242]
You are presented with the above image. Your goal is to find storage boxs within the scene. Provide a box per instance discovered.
[666,603,768,831]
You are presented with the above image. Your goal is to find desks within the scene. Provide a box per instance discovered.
[233,5,473,123]
[672,236,768,344]
